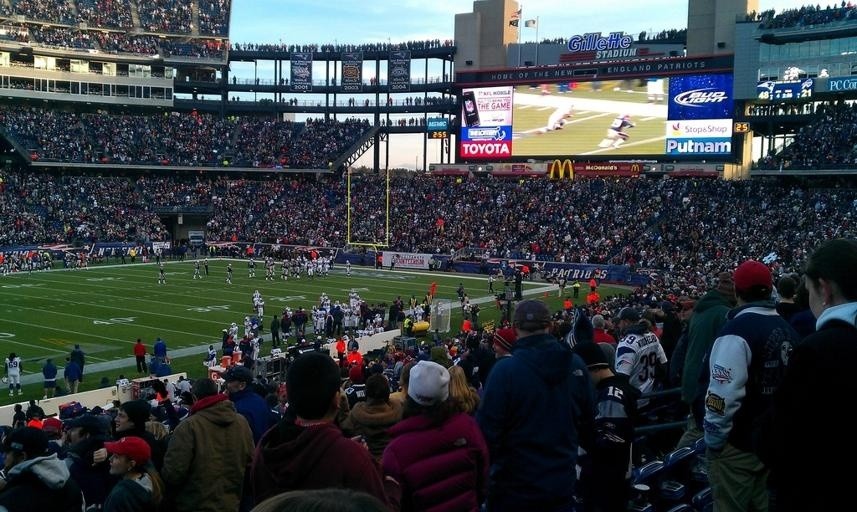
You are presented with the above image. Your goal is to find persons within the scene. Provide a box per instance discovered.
[0,2,856,511]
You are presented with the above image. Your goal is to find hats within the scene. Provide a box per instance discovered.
[217,366,254,382]
[714,272,735,296]
[121,399,152,429]
[407,359,451,406]
[0,412,112,453]
[513,299,553,322]
[612,307,641,320]
[735,261,772,291]
[493,327,517,353]
[103,436,152,465]
[571,342,609,368]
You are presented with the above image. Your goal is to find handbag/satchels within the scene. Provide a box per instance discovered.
[58,402,90,420]
